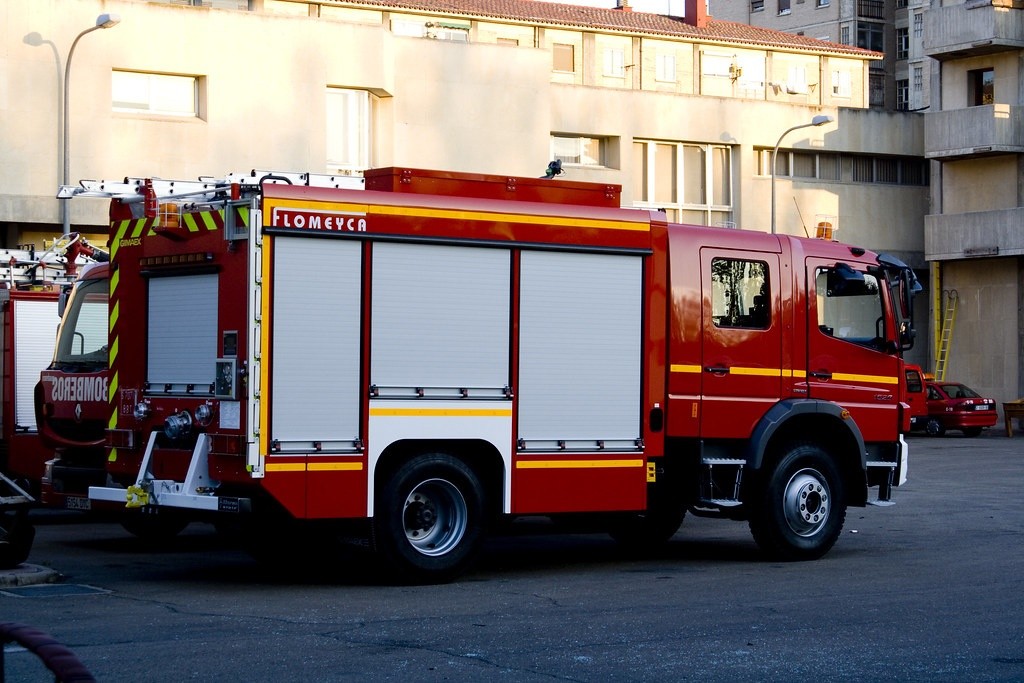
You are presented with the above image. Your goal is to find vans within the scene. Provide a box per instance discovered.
[905,363,929,433]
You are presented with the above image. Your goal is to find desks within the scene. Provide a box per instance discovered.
[1002,399,1024,437]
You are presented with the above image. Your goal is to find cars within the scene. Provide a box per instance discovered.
[907,373,999,438]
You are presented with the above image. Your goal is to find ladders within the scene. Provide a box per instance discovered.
[55,166,370,213]
[0,246,97,288]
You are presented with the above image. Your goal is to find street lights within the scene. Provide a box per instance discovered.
[770,113,836,234]
[60,11,122,240]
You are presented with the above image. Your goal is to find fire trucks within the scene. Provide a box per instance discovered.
[1,168,923,586]
